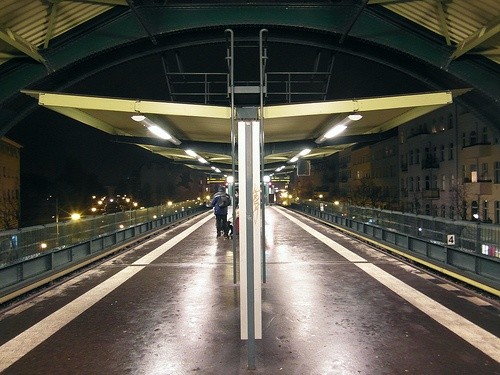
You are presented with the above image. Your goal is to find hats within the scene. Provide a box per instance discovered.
[218,187,225,192]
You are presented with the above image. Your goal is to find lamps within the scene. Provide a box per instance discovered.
[276,114,362,172]
[131,115,221,173]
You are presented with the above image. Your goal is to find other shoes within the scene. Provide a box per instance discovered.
[216,235,221,238]
[224,235,229,239]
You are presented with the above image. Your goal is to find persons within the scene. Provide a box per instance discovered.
[210,185,231,239]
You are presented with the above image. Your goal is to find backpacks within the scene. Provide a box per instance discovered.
[217,193,231,208]
